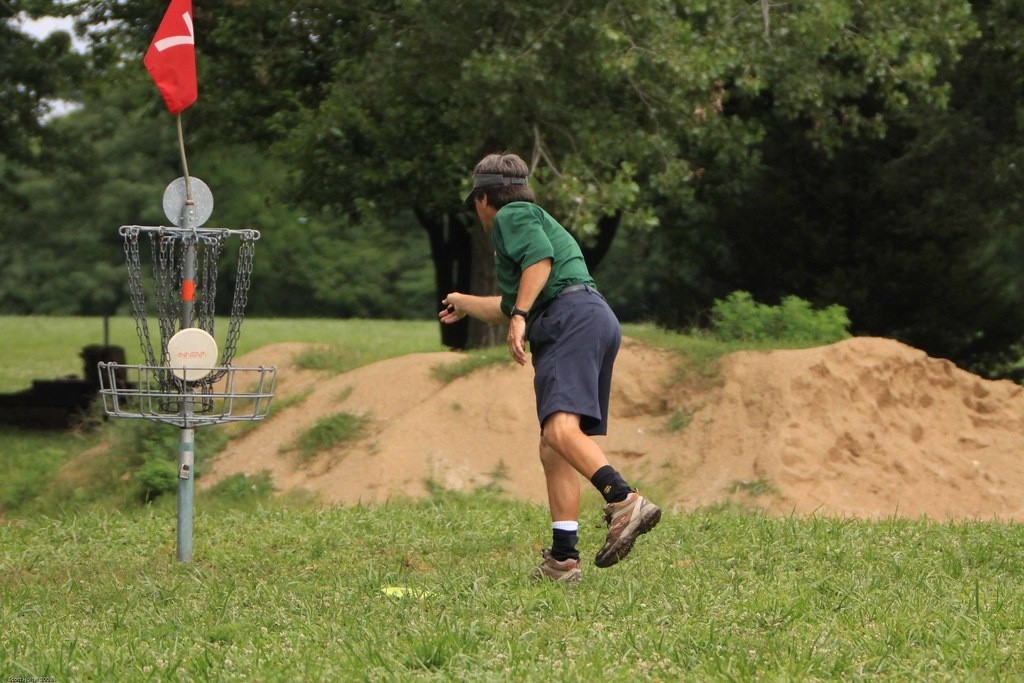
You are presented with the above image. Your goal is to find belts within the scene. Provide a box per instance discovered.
[557,284,603,300]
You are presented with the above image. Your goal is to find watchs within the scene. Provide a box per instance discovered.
[510,305,529,319]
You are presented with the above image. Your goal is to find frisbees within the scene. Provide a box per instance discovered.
[382,587,434,600]
[166,328,218,381]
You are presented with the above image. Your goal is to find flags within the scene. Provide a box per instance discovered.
[143,0,198,115]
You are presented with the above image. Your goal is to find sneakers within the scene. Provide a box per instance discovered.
[594,488,661,568]
[531,549,582,584]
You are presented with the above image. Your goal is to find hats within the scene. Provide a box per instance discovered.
[464,174,528,206]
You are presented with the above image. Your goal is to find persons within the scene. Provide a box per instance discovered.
[438,154,662,583]
